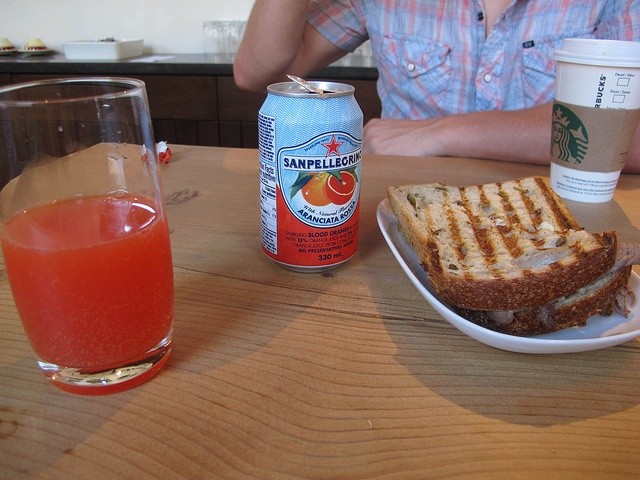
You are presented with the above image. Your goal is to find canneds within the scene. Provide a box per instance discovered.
[257,74,364,274]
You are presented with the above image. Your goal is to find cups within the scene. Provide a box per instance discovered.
[549,36,640,203]
[0,77,174,395]
[200,20,248,57]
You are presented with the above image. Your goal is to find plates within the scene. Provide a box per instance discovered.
[376,196,639,354]
[17,49,54,55]
[1,49,17,53]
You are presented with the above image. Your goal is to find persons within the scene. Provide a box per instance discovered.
[233,1,640,175]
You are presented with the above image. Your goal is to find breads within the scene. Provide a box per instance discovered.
[452,241,640,336]
[386,176,617,310]
